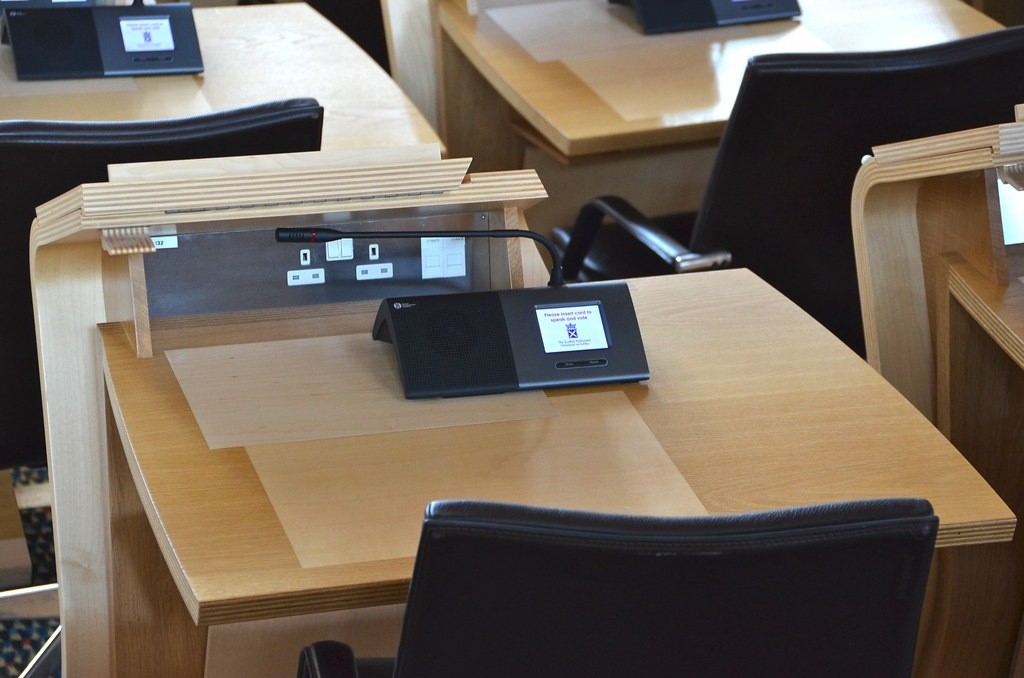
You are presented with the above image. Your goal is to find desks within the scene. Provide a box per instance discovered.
[919,236,1024,678]
[431,0,1024,273]
[92,270,1018,678]
[0,2,445,183]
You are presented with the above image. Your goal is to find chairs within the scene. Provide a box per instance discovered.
[0,96,321,471]
[298,498,937,678]
[546,23,1024,357]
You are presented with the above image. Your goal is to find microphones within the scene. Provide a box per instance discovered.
[275,226,564,288]
